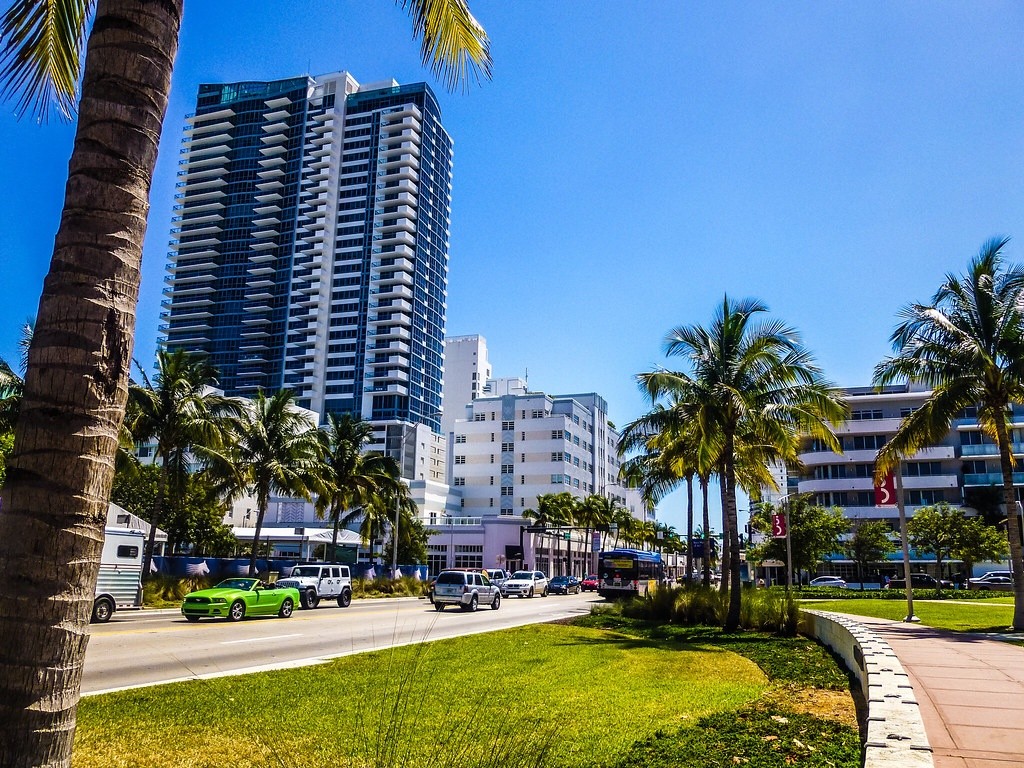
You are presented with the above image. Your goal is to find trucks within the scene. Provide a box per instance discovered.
[89,525,147,622]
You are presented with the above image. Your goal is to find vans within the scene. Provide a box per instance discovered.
[441,568,509,592]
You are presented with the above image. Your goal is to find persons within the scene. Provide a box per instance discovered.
[885,574,889,584]
[955,576,959,589]
[892,573,897,579]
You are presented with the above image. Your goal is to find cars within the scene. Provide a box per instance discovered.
[547,575,582,596]
[810,576,848,588]
[970,576,1015,592]
[581,574,600,593]
[676,569,723,587]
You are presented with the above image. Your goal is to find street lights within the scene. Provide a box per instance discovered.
[585,483,617,576]
[392,412,446,581]
[243,509,260,528]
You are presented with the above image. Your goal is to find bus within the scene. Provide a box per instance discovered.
[596,548,667,603]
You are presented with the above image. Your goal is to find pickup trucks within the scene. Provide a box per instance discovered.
[888,572,954,590]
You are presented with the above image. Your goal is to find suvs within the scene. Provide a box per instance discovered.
[274,561,354,609]
[431,571,501,613]
[501,570,549,599]
[964,570,1016,590]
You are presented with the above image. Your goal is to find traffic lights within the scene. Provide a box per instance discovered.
[663,532,674,538]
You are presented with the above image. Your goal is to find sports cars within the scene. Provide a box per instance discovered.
[181,577,301,623]
